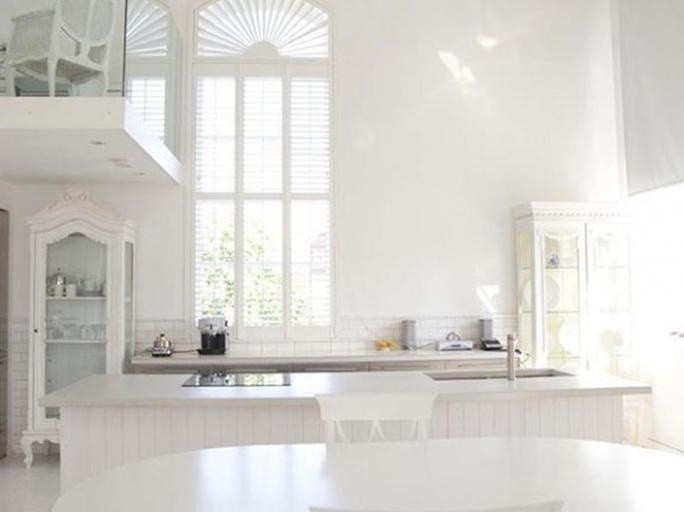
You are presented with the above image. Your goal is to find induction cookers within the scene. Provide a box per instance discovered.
[181,372,292,388]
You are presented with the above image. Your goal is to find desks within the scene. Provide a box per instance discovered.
[48,438,684,512]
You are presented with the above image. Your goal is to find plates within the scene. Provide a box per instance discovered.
[557,317,577,346]
[524,272,561,310]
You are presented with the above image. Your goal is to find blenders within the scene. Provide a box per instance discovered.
[477,308,503,352]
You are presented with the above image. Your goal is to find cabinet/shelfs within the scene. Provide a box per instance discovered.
[22,197,136,467]
[515,200,638,438]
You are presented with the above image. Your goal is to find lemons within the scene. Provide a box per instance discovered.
[375,339,397,348]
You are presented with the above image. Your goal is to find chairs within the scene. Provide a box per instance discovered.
[317,395,434,442]
[7,0,117,97]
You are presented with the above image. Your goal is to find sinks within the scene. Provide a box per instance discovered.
[422,368,577,381]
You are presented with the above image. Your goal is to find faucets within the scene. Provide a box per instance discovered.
[507,332,522,381]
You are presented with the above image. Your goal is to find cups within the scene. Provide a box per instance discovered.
[64,284,76,297]
[82,279,96,290]
[401,318,416,353]
[46,318,105,340]
[54,285,64,297]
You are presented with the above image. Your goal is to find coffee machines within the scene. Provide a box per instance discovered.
[197,312,228,353]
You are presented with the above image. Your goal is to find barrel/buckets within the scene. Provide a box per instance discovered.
[198,316,227,351]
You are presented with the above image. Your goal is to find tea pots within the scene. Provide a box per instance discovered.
[46,272,65,296]
